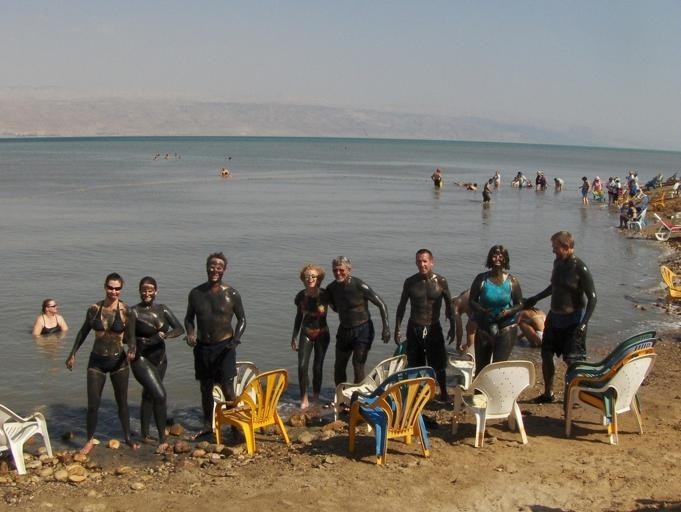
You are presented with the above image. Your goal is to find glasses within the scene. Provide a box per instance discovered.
[47,305,57,307]
[304,273,318,278]
[106,286,121,291]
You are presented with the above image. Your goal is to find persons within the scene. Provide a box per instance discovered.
[228,156,232,160]
[524,179,533,189]
[627,168,642,197]
[535,170,547,192]
[122,277,185,454]
[31,299,69,339]
[645,172,663,191]
[515,171,529,189]
[554,177,565,192]
[592,176,605,201]
[578,176,590,206]
[511,176,521,190]
[517,296,548,348]
[445,289,483,354]
[65,273,142,455]
[152,150,182,160]
[432,167,443,190]
[454,181,479,192]
[184,254,247,446]
[494,170,501,193]
[325,256,391,413]
[616,178,622,200]
[525,232,598,409]
[468,246,525,396]
[482,178,494,204]
[394,249,457,404]
[221,167,228,175]
[291,265,340,409]
[608,177,616,206]
[618,200,642,230]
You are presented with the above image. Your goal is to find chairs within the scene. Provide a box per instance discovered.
[660,265,681,304]
[211,361,259,430]
[215,370,293,453]
[334,355,407,421]
[667,182,680,199]
[0,404,52,473]
[654,215,681,240]
[454,361,536,448]
[564,331,658,446]
[348,366,436,464]
[628,207,645,231]
[650,190,665,213]
